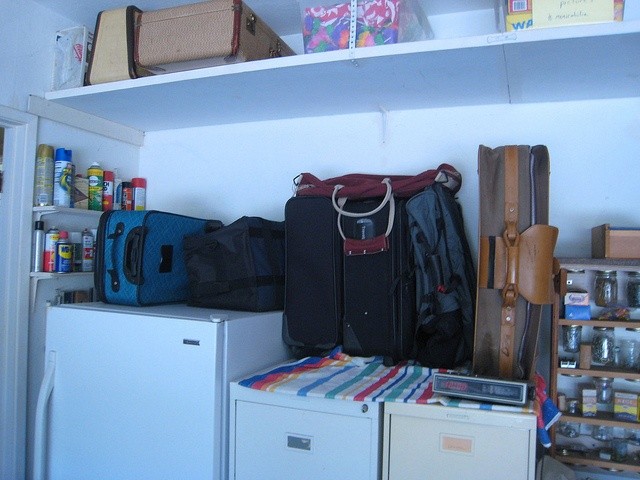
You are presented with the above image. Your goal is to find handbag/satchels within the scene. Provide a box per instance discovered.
[470,144,559,384]
[181,214,287,312]
[293,163,463,257]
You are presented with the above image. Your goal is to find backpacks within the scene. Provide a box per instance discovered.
[406,185,477,369]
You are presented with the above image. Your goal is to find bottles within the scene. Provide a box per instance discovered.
[33,144,55,207]
[56,231,74,273]
[30,219,45,271]
[610,347,622,368]
[132,178,148,211]
[87,162,103,211]
[103,171,114,212]
[595,270,619,307]
[113,168,123,210]
[592,326,615,363]
[122,181,133,211]
[52,148,72,208]
[68,232,82,272]
[44,225,60,273]
[81,228,94,272]
[593,376,614,404]
[561,323,582,354]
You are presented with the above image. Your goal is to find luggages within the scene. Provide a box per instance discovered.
[92,209,224,308]
[341,200,406,367]
[282,197,341,359]
[135,1,298,76]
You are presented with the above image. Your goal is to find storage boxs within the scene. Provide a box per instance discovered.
[298,0,400,53]
[504,1,623,32]
[592,222,640,257]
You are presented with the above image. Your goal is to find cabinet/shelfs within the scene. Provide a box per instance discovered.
[28,204,112,304]
[552,259,640,474]
[383,401,536,480]
[230,383,381,480]
[44,19,640,134]
